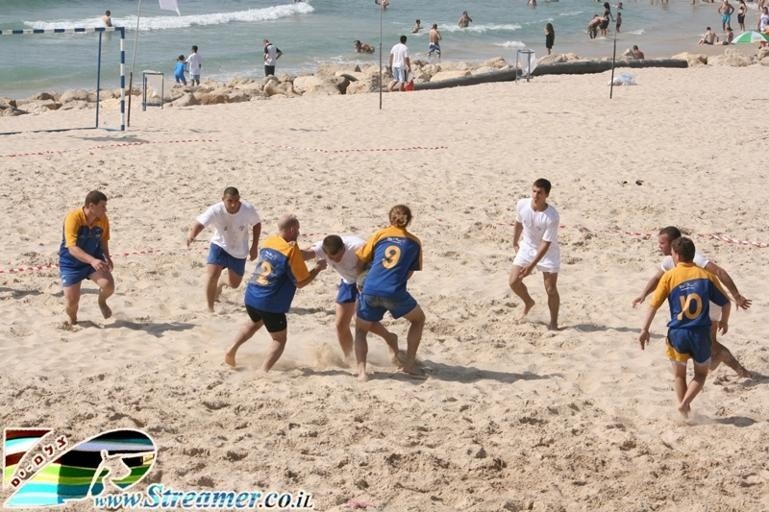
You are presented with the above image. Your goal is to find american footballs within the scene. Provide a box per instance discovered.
[355,269,370,293]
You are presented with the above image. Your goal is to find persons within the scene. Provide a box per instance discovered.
[599,2,615,37]
[761,20,769,48]
[174,55,187,86]
[412,20,423,33]
[640,237,731,420]
[458,11,472,28]
[617,2,623,9]
[225,218,327,375]
[427,23,441,57]
[616,13,622,32]
[288,235,398,359]
[758,0,765,11]
[353,39,374,54]
[186,188,261,313]
[58,190,115,327]
[624,49,633,60]
[262,40,283,76]
[510,179,560,330]
[632,46,644,60]
[737,0,747,32]
[588,15,608,39]
[528,0,536,6]
[698,27,719,46]
[661,0,714,5]
[388,35,411,91]
[355,205,425,382]
[544,23,555,55]
[184,45,202,86]
[723,28,733,45]
[718,0,735,31]
[102,10,112,27]
[375,0,389,8]
[631,227,751,377]
[757,7,769,49]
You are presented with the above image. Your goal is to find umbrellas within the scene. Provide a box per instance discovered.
[731,29,769,45]
